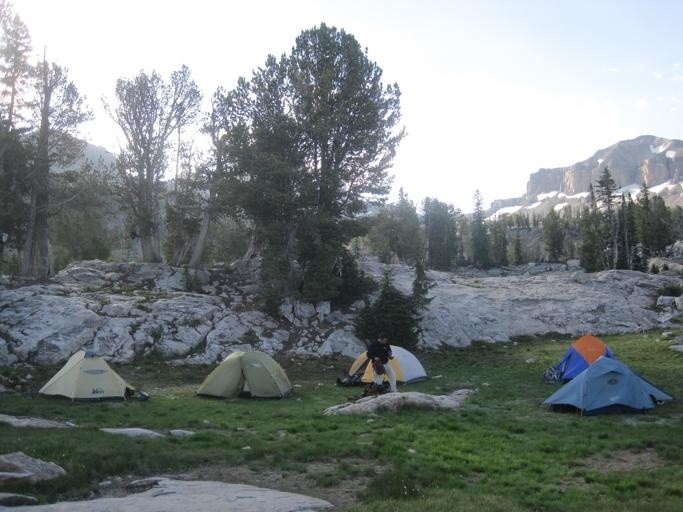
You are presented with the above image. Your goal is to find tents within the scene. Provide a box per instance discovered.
[37,348,149,403]
[340,343,428,386]
[543,335,616,383]
[194,351,293,400]
[539,356,676,416]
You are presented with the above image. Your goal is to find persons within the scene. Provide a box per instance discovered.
[364,331,399,393]
